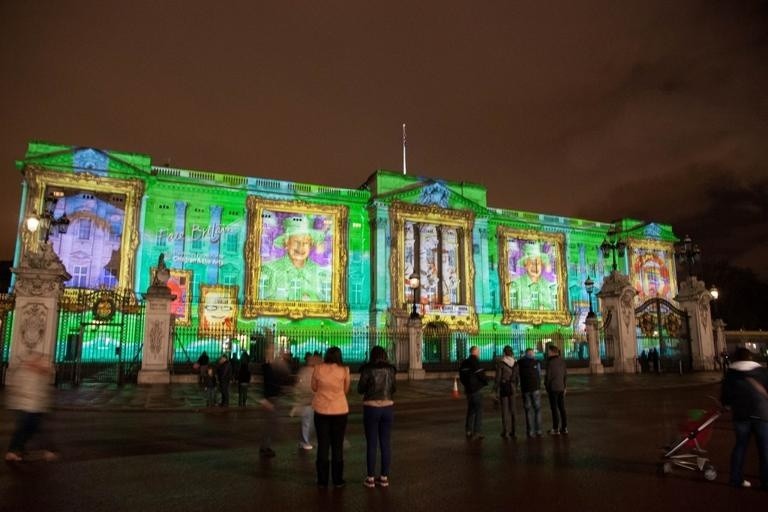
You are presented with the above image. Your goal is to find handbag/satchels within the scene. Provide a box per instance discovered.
[735,376,768,417]
[500,381,512,398]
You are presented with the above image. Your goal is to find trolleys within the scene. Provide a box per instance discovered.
[662,406,731,482]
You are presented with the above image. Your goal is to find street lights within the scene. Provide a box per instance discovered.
[600,238,626,271]
[676,234,701,276]
[585,276,594,313]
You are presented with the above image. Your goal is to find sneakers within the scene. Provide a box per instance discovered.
[336,480,346,487]
[501,427,568,438]
[259,448,275,456]
[466,431,483,439]
[6,452,22,461]
[364,480,376,487]
[742,480,751,487]
[297,444,312,449]
[376,475,389,486]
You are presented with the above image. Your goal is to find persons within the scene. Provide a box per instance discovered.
[287,350,320,387]
[254,215,332,303]
[719,346,767,491]
[541,344,569,436]
[2,345,62,464]
[457,345,490,440]
[291,350,353,452]
[310,345,353,490]
[712,346,768,373]
[514,348,545,437]
[197,351,253,408]
[490,345,521,439]
[45,193,124,290]
[638,347,660,375]
[356,345,398,488]
[251,341,292,462]
[507,242,559,311]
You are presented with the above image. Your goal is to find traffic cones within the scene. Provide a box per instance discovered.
[453,377,461,398]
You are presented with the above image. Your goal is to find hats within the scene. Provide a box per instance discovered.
[273,216,326,249]
[515,243,550,268]
